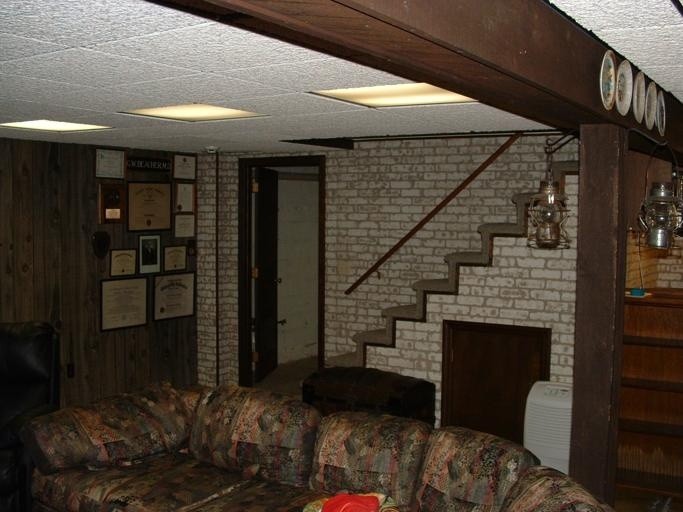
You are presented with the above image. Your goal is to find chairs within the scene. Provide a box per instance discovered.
[0,320,62,512]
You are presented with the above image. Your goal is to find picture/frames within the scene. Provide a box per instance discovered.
[92,145,129,181]
[96,275,149,334]
[171,152,199,182]
[150,269,197,323]
[171,180,197,215]
[107,247,139,277]
[136,233,159,275]
[125,178,173,234]
[160,244,189,274]
[171,211,197,242]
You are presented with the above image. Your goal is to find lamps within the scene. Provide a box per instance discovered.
[522,127,577,250]
[627,125,682,250]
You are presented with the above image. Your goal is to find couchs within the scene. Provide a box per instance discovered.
[15,385,614,512]
